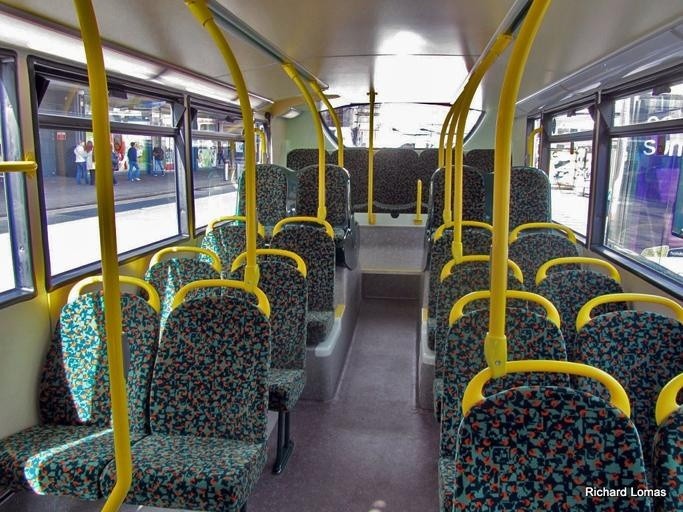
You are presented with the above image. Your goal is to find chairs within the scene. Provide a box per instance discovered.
[0,274,161,500]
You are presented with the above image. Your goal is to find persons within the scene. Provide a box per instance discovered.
[73,139,164,186]
